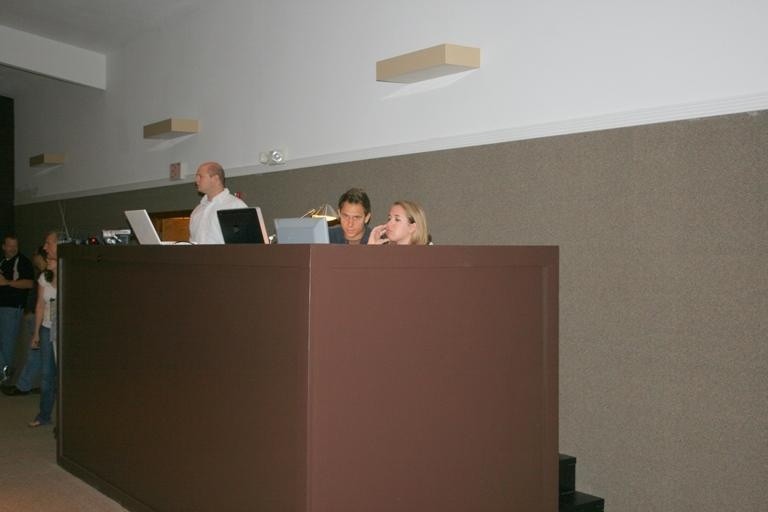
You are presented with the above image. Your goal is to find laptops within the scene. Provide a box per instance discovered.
[124,209,176,245]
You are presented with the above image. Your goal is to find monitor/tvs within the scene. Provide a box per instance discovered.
[216,208,270,244]
[274,218,330,245]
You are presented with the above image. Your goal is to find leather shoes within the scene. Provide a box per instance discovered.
[31,387,41,395]
[1,384,29,396]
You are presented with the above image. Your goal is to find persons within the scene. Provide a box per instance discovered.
[329,187,374,245]
[187,162,250,246]
[367,200,434,245]
[0,231,64,427]
